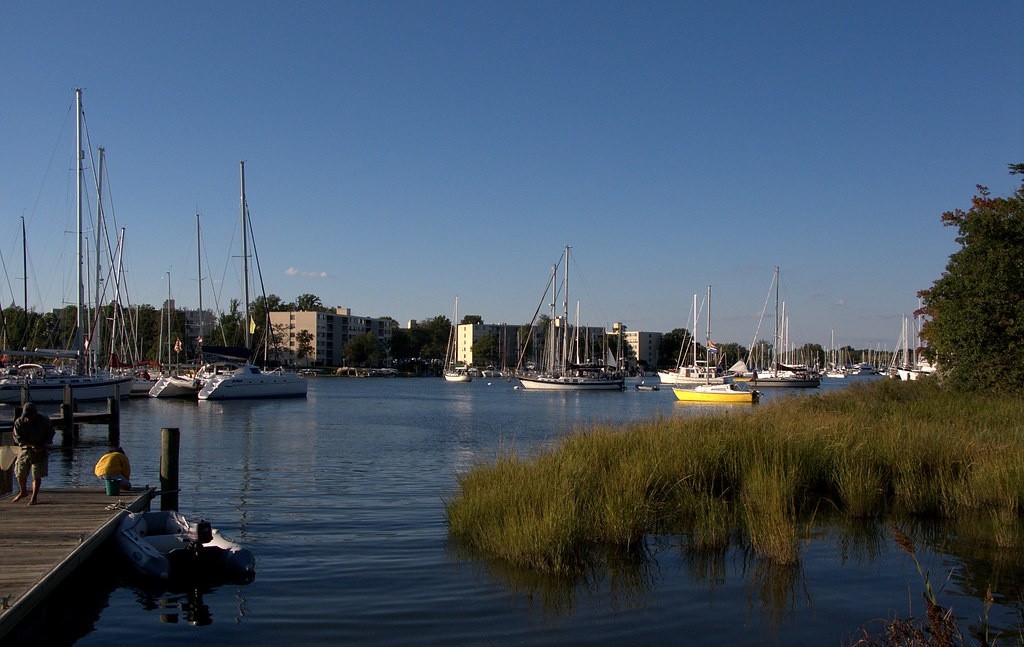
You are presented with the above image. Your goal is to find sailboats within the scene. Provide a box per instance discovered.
[1,86,310,405]
[440,243,944,392]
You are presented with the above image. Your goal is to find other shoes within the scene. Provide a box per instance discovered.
[13,494,37,505]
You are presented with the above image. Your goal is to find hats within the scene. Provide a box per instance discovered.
[21,402,36,418]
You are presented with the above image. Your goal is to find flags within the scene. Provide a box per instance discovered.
[707,339,718,353]
[249,315,256,335]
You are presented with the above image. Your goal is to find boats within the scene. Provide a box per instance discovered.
[115,509,256,588]
[669,383,762,403]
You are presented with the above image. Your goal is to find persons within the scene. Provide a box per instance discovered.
[12,402,56,505]
[53,357,62,366]
[94,447,131,489]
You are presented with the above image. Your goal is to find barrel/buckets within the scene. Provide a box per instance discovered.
[105,479,122,496]
